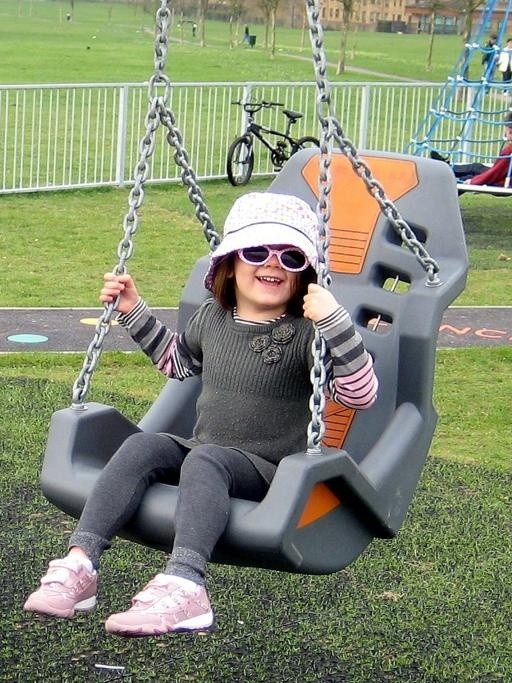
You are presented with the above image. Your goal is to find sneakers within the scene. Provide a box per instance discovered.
[23,556,98,620]
[431,150,449,164]
[103,573,214,638]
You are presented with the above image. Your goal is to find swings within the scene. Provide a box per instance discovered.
[39,0,469,575]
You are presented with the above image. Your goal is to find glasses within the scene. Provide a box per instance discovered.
[237,245,311,273]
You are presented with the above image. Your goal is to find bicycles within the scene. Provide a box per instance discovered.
[227,100,320,187]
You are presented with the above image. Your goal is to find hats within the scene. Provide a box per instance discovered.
[202,191,325,293]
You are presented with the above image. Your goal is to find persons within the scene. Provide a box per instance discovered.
[66,14,71,20]
[431,111,512,197]
[192,23,197,37]
[24,192,378,638]
[481,34,512,95]
[241,24,250,44]
